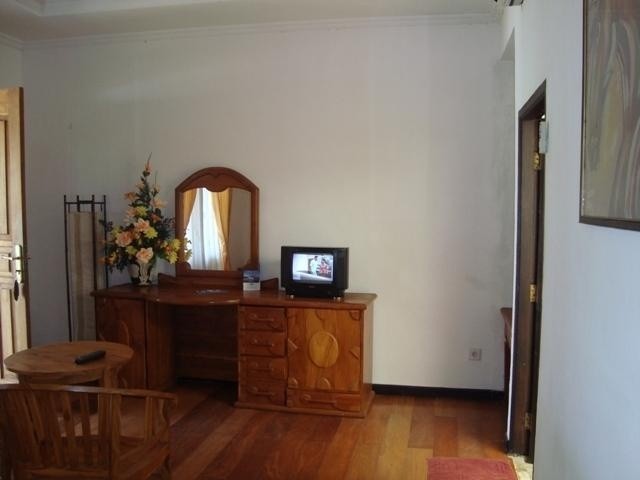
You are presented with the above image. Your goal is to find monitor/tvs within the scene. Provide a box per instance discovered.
[280,245,349,300]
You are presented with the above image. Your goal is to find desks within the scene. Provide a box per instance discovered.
[90,282,376,418]
[500,307,512,431]
[4,340,134,437]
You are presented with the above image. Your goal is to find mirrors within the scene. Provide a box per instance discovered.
[175,166,258,288]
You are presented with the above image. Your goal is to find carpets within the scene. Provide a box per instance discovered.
[426,456,518,480]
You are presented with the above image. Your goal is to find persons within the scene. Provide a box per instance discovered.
[319,258,329,275]
[310,256,318,276]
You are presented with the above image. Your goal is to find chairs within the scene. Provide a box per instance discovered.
[0,383,178,480]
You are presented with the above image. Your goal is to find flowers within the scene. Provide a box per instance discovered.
[97,153,181,273]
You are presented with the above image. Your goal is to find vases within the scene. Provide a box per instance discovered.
[136,261,154,286]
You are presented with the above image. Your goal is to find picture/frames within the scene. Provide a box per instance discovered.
[579,0,640,233]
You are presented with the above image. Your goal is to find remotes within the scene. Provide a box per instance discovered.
[75,349,105,365]
